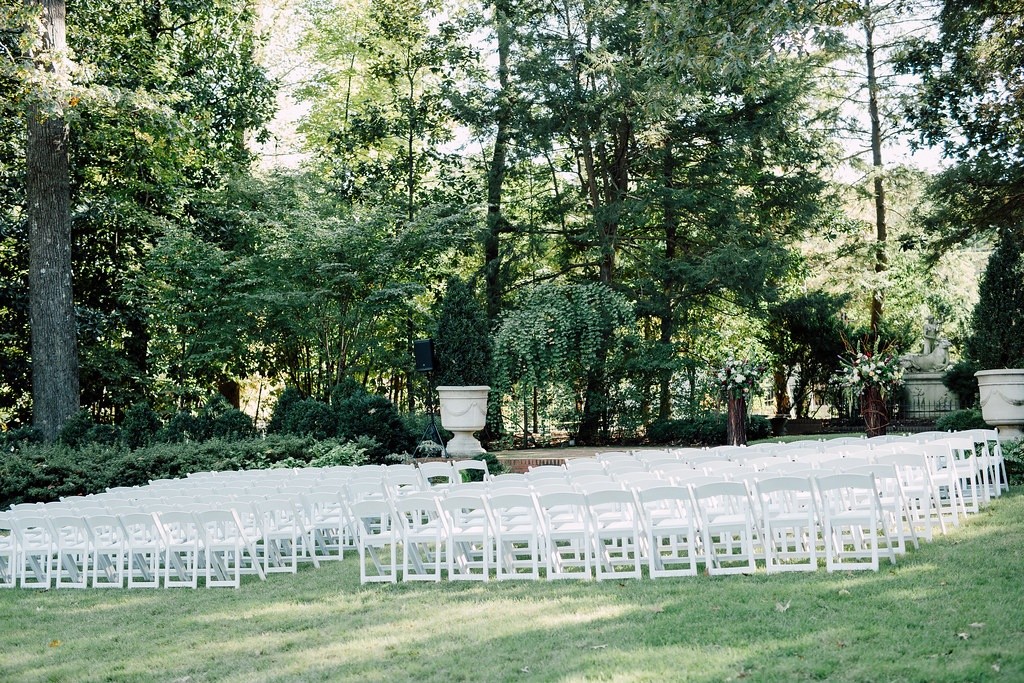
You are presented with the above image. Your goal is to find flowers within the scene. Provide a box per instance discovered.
[830,331,907,389]
[709,343,766,400]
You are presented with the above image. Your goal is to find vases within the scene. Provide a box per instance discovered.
[435,272,492,457]
[961,224,1024,440]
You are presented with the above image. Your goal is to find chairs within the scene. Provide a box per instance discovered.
[0,427,1013,589]
